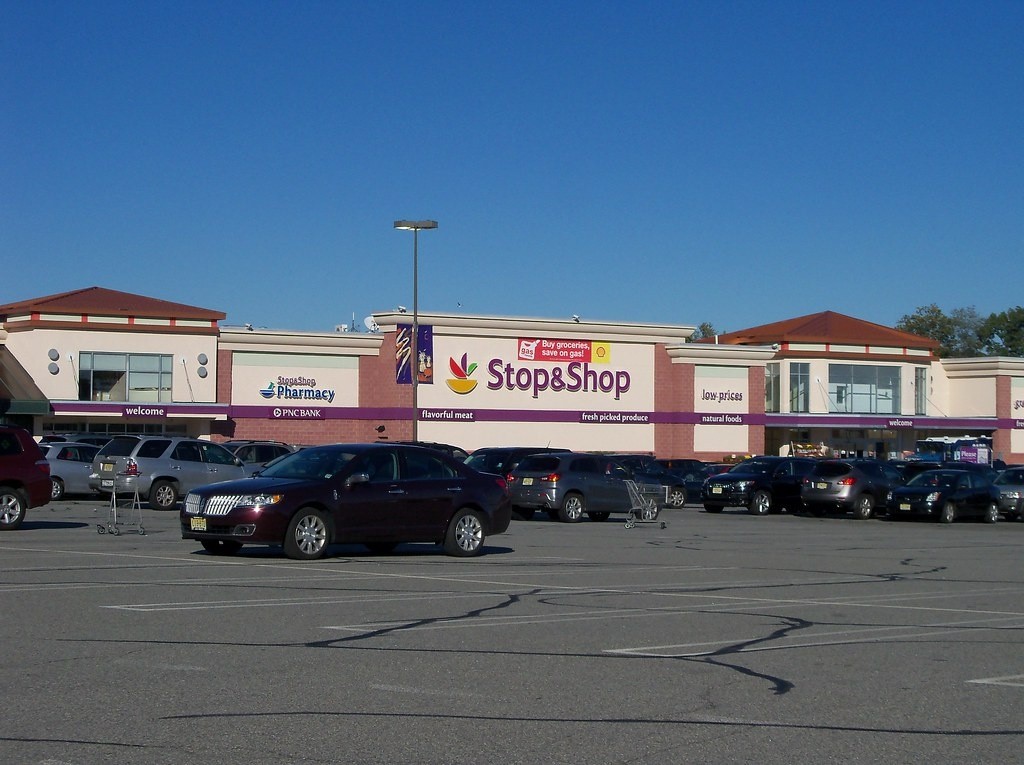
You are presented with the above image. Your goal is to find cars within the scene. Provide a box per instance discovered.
[176,443,514,561]
[371,445,1023,588]
[36,442,103,501]
[89,434,250,512]
[206,438,297,479]
[40,432,113,461]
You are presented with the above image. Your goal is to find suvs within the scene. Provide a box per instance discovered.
[0,423,54,532]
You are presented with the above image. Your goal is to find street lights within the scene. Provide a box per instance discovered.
[392,219,438,441]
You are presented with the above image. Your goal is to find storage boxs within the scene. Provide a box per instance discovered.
[102,392,110,401]
[93,390,101,401]
